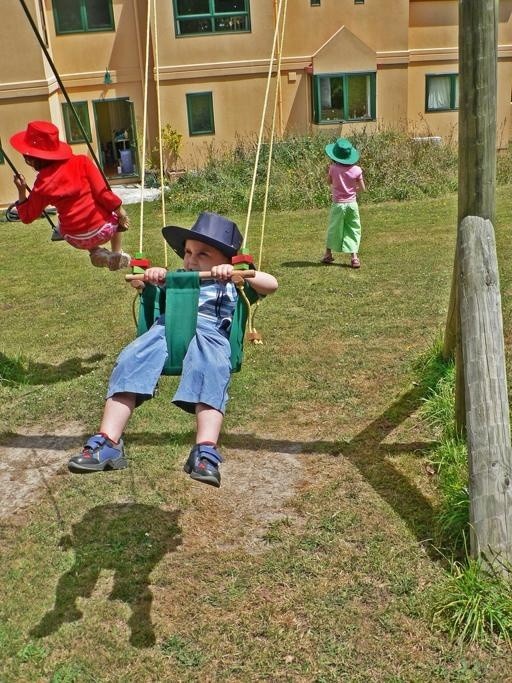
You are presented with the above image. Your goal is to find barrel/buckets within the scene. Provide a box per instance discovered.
[118,166,122,174]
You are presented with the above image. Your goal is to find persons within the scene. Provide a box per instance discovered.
[8,120,132,270]
[65,211,280,488]
[319,137,367,268]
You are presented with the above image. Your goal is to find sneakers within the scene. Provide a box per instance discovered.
[321,254,334,263]
[351,258,360,267]
[68,432,127,471]
[184,443,222,487]
[110,253,131,271]
[90,247,112,267]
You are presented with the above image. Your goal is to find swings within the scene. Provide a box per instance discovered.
[0,0,128,242]
[125,0,288,376]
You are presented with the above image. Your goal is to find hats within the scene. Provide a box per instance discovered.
[162,211,244,258]
[10,121,73,161]
[326,138,360,165]
